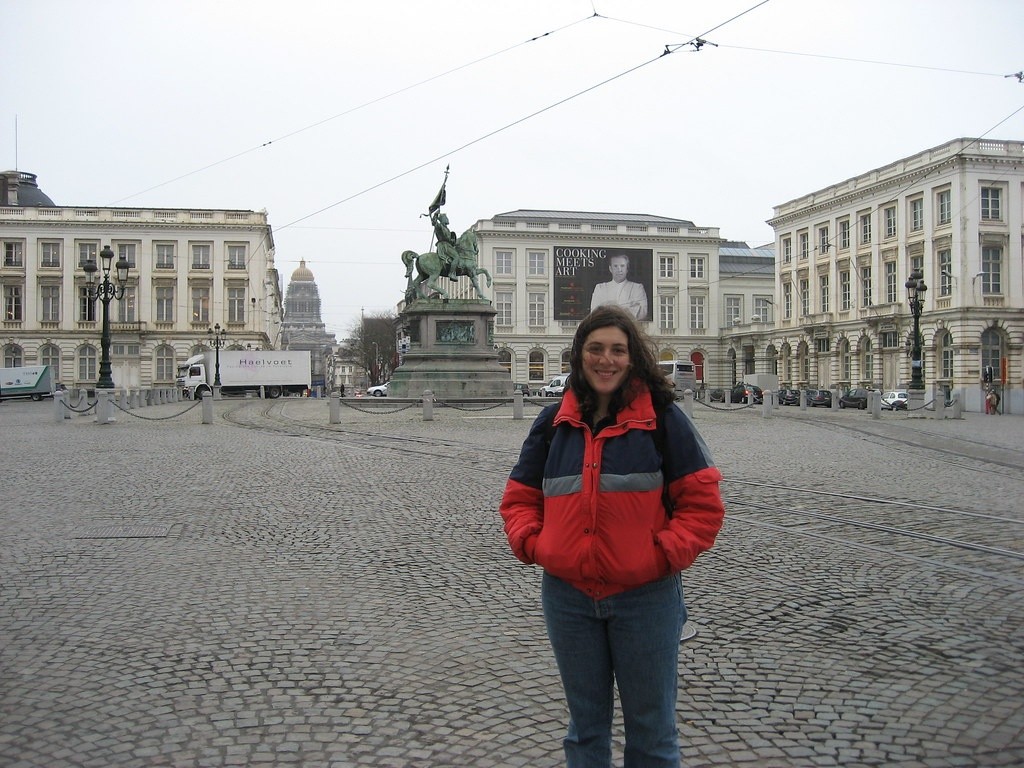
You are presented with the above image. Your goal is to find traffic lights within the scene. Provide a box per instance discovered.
[984,370,989,382]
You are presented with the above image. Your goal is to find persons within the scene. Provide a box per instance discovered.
[590,254,647,321]
[985,389,1001,415]
[340,383,345,397]
[499,305,725,768]
[431,210,459,281]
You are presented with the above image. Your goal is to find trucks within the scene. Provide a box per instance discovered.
[175,349,312,400]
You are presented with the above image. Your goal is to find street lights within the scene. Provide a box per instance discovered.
[904,267,927,417]
[82,243,131,423]
[372,342,378,385]
[207,323,229,400]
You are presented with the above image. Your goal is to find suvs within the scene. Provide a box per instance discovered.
[514,382,530,397]
[731,382,763,405]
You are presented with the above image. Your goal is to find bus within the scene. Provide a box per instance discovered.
[656,360,697,399]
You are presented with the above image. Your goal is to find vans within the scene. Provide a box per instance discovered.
[538,375,567,397]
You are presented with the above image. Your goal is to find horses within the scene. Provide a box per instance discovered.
[402,230,491,298]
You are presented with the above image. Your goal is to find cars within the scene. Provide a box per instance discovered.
[710,387,732,403]
[779,390,801,406]
[806,389,832,408]
[56,383,67,391]
[839,387,870,410]
[881,392,908,410]
[367,382,389,397]
